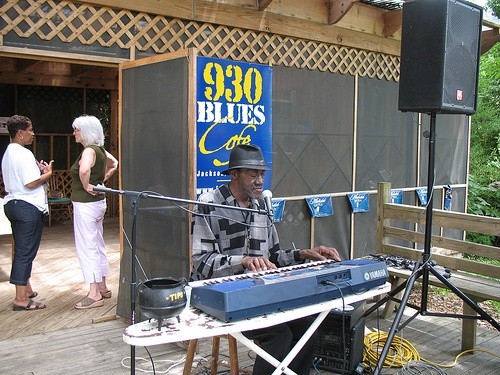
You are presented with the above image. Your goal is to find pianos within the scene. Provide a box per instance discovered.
[187,258,390,324]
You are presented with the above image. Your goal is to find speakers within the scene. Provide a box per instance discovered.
[399,0,484,116]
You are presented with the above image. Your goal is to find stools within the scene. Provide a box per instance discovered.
[183,335,238,375]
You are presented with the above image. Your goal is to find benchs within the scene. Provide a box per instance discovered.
[377,181,500,355]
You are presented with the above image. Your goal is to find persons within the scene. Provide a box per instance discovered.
[1,116,55,311]
[188,145,342,375]
[68,115,119,310]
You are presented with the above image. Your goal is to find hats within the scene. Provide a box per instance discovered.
[220,144,272,175]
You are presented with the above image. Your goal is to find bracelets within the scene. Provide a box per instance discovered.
[294,249,307,263]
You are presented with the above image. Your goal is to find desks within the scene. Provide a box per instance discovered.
[122,282,391,375]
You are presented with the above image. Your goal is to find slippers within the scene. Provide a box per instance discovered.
[74,296,105,309]
[29,291,38,298]
[13,297,46,311]
[101,290,111,298]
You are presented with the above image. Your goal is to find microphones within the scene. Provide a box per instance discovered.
[262,190,274,223]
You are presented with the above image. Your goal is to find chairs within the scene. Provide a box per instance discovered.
[46,174,72,228]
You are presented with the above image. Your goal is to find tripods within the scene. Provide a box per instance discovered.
[361,111,500,375]
